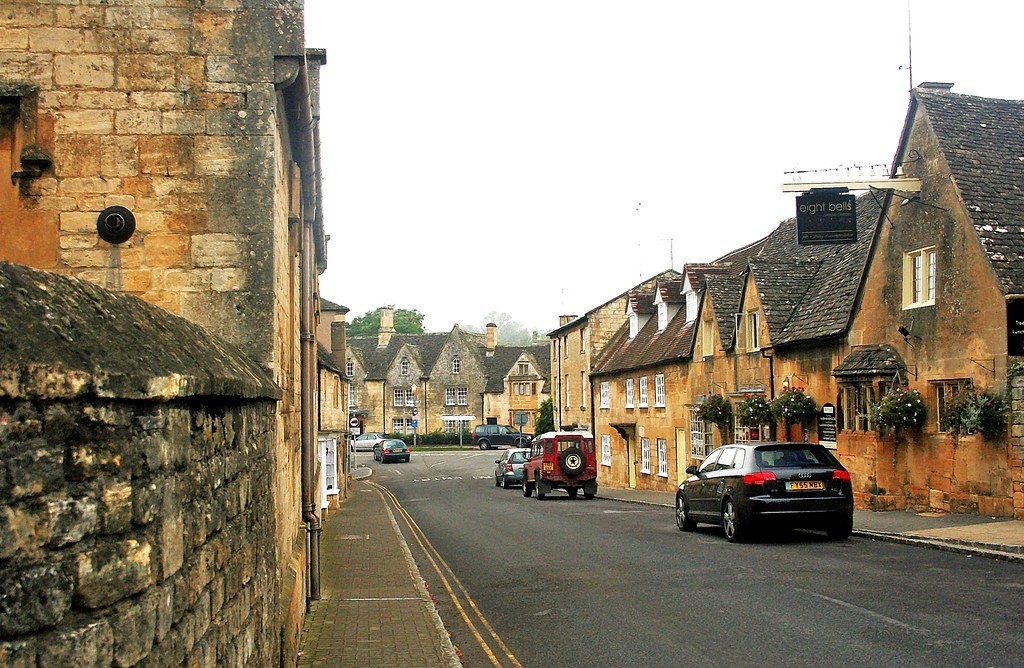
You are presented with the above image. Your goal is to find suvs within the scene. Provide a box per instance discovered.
[471,424,534,450]
[522,432,598,500]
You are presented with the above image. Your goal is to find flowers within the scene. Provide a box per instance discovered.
[734,391,773,427]
[766,383,817,427]
[938,385,1008,449]
[869,384,928,466]
[692,391,735,445]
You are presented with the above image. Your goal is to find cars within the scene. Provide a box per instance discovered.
[676,442,853,542]
[374,440,411,464]
[495,449,539,489]
[350,433,406,452]
